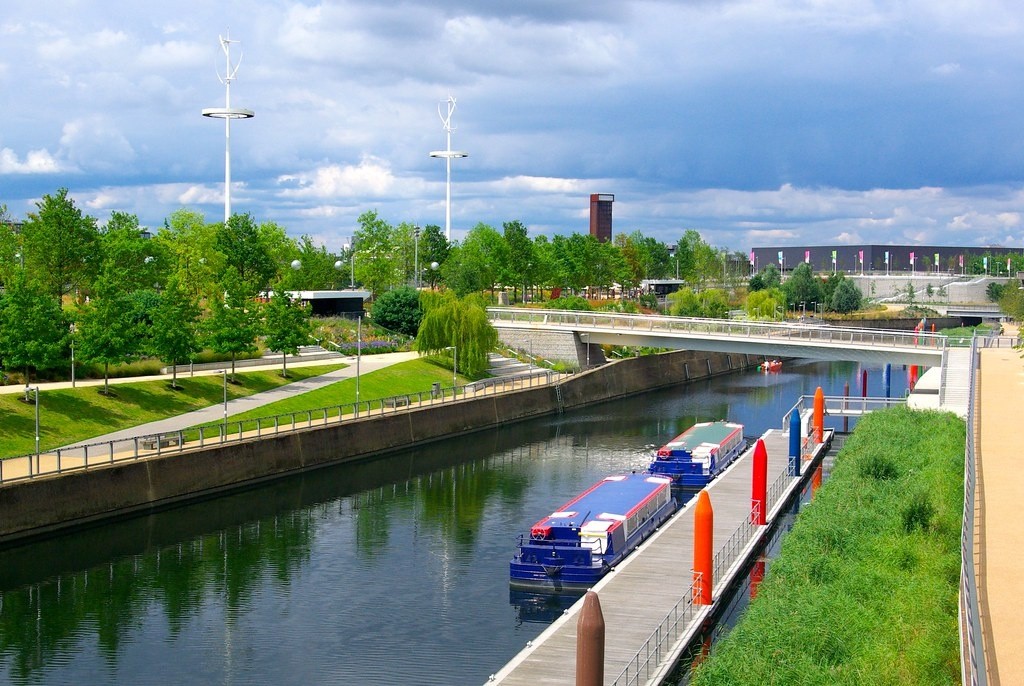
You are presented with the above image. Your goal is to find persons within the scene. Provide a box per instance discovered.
[801,314,806,322]
[773,357,779,364]
[763,359,771,370]
[797,314,801,323]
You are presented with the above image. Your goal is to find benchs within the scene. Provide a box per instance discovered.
[384,398,411,408]
[139,435,187,450]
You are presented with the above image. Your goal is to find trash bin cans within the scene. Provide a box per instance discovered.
[432,383,441,396]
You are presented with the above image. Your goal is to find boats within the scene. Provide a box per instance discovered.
[508,469,677,597]
[650,419,749,490]
